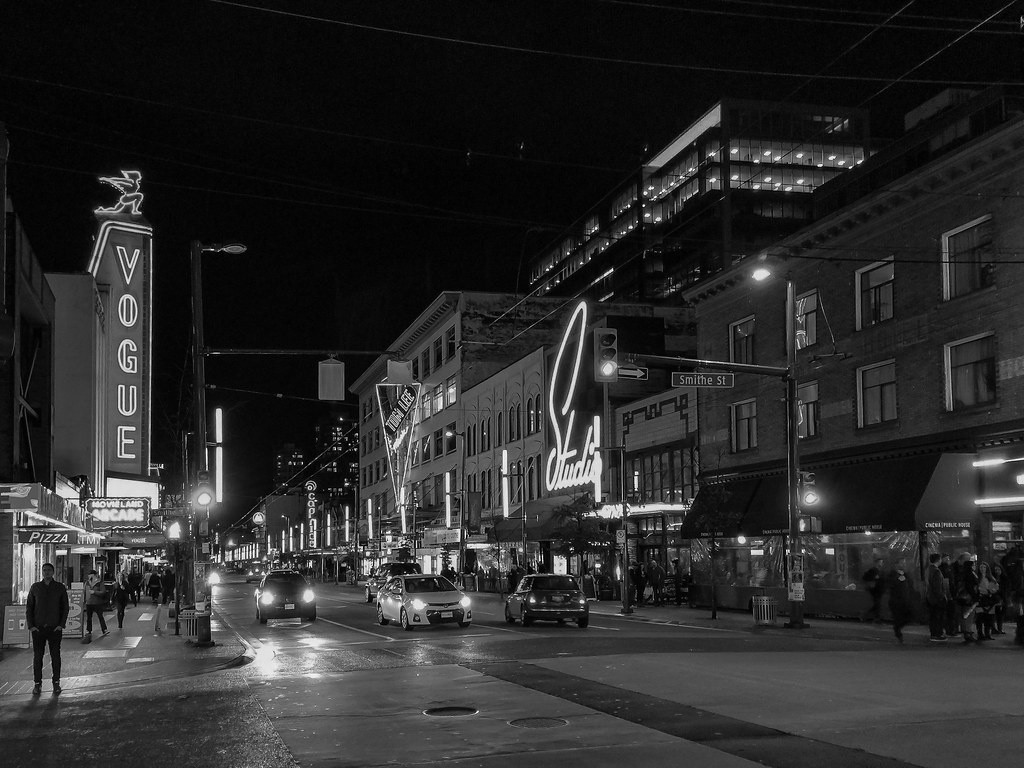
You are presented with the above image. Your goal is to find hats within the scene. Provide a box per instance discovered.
[88,570,97,574]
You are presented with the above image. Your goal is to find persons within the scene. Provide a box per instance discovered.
[85,570,110,636]
[925,552,1024,646]
[629,560,666,608]
[580,560,594,576]
[672,560,683,607]
[538,562,548,573]
[478,566,484,574]
[300,566,312,576]
[889,557,914,641]
[463,563,468,571]
[26,563,70,694]
[507,562,533,594]
[370,566,375,575]
[488,566,497,589]
[129,568,175,607]
[110,572,130,628]
[863,559,885,625]
[440,565,457,585]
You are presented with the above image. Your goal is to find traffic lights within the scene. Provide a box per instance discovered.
[193,470,217,507]
[594,326,617,381]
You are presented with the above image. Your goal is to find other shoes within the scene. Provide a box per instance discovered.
[947,634,962,637]
[965,632,976,641]
[85,633,91,636]
[103,630,110,635]
[991,630,999,634]
[53,683,61,693]
[929,635,948,642]
[998,629,1006,634]
[33,683,42,694]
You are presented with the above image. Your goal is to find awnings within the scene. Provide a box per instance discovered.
[487,490,588,543]
[680,452,981,538]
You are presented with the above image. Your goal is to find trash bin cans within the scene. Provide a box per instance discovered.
[752,595,778,626]
[463,575,478,594]
[177,609,198,639]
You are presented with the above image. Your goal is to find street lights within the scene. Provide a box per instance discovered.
[190,239,248,470]
[445,428,467,574]
[749,268,808,628]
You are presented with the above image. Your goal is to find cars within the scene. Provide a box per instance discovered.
[375,572,472,631]
[363,563,419,605]
[253,568,317,623]
[501,573,591,629]
[226,558,266,584]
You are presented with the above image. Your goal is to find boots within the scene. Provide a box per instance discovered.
[977,623,988,640]
[985,625,995,640]
